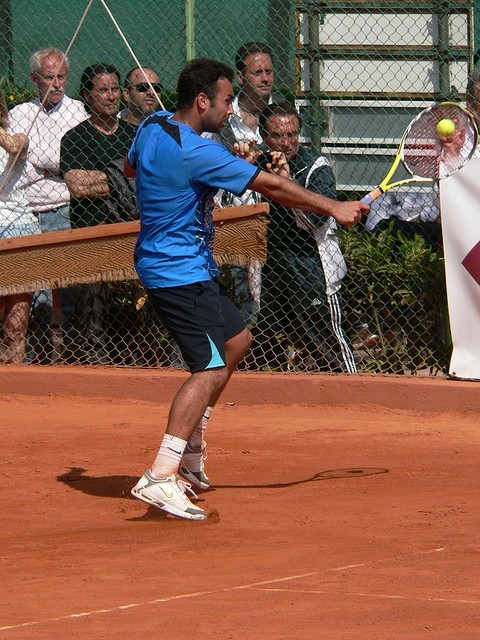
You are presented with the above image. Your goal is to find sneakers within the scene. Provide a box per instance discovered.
[131,470,207,520]
[178,442,209,489]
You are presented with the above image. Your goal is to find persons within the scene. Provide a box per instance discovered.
[116,66,163,126]
[59,63,140,368]
[0,88,43,366]
[7,46,95,366]
[233,101,358,374]
[200,41,287,309]
[123,57,371,522]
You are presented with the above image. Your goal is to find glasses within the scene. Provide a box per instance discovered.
[132,82,163,93]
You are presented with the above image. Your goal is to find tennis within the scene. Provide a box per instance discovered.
[436,118,455,136]
